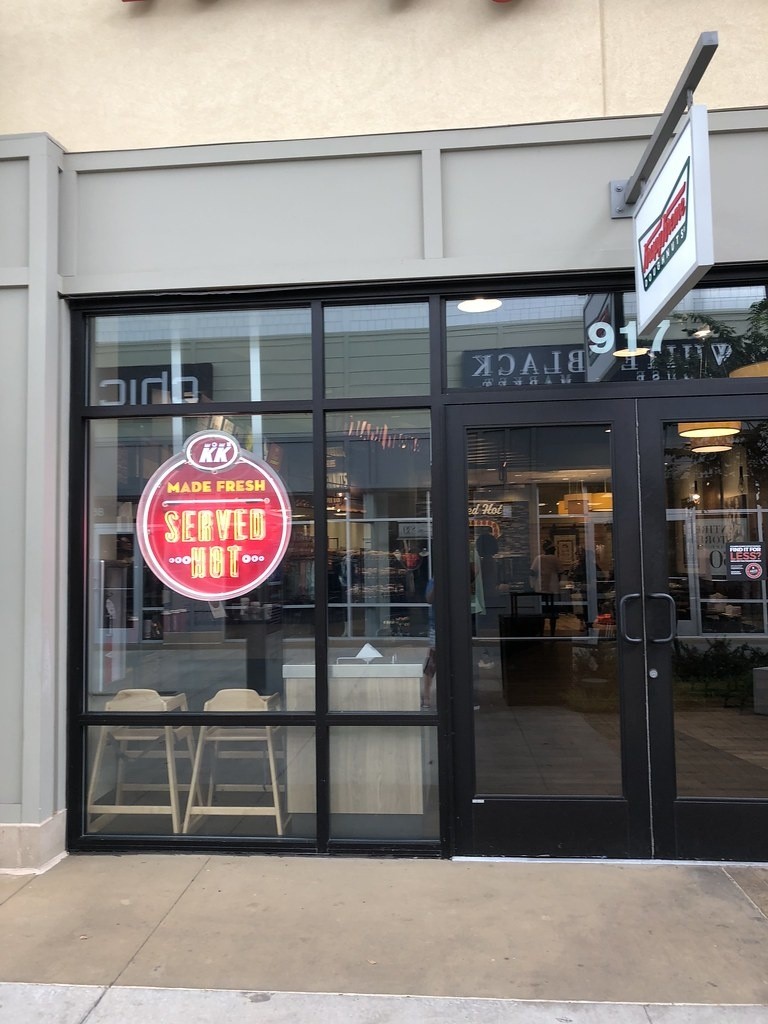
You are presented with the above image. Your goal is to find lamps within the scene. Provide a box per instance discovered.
[677,421,742,438]
[557,477,613,514]
[728,361,768,377]
[690,434,734,453]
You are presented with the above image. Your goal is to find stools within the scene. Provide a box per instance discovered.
[86,688,291,836]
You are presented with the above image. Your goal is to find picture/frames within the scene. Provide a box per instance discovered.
[550,529,579,571]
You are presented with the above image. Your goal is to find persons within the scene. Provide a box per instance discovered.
[421,577,480,711]
[529,539,565,637]
[470,533,500,668]
[573,548,605,637]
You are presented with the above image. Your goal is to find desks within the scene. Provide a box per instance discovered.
[278,646,432,834]
